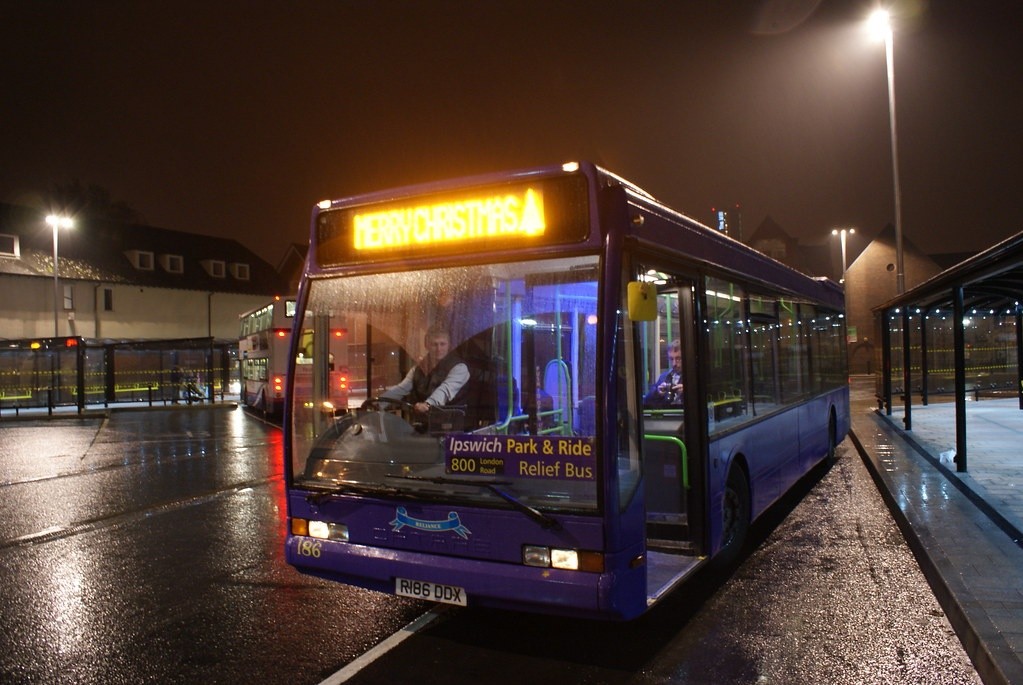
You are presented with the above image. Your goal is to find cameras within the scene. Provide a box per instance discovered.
[663,383,677,403]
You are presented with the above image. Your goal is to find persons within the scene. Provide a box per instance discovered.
[645,340,683,409]
[371,325,480,438]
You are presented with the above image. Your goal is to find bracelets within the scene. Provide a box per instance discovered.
[424,403,430,410]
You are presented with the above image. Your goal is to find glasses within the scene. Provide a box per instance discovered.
[668,356,682,364]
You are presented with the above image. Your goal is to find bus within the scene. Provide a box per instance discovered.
[281,157,851,624]
[236,292,349,422]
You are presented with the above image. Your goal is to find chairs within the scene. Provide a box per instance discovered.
[459,336,487,424]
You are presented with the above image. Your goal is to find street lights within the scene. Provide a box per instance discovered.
[829,228,856,279]
[867,7,905,296]
[45,215,77,403]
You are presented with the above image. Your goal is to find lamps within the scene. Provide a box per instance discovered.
[63,285,73,310]
